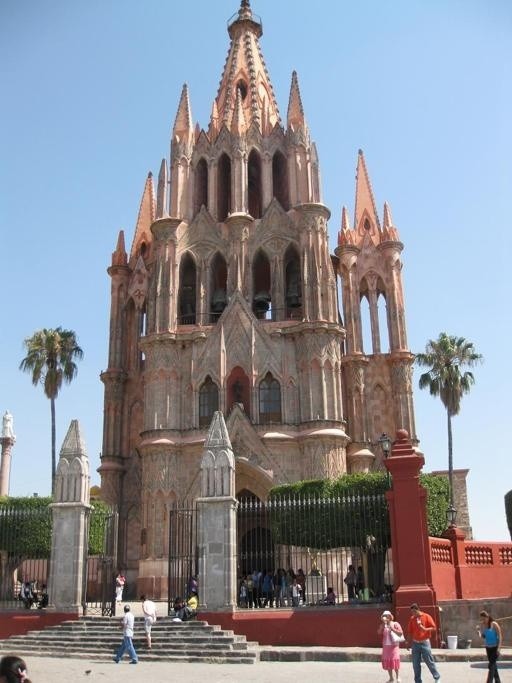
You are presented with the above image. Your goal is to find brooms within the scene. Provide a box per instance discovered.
[439,610,447,648]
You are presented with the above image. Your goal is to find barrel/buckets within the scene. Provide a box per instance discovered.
[447,636,458,649]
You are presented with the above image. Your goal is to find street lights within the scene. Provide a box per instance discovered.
[444,503,458,525]
[378,430,393,488]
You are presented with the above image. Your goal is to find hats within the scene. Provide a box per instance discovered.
[381,610,394,622]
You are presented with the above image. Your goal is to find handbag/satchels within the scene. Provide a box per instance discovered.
[391,631,406,643]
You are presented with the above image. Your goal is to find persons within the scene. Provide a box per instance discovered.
[37,584,48,608]
[113,604,139,663]
[403,602,440,683]
[140,594,157,650]
[120,574,126,591]
[473,610,502,683]
[376,610,405,683]
[1,655,31,683]
[115,572,127,606]
[18,581,34,609]
[171,564,394,621]
